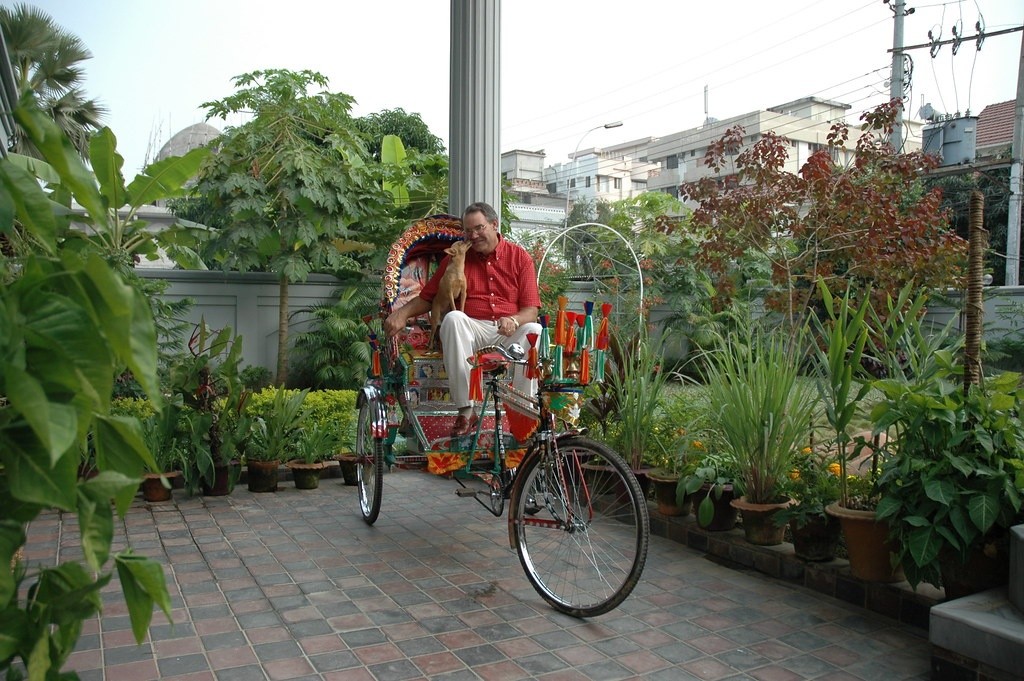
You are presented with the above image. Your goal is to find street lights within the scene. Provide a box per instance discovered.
[561,120,622,267]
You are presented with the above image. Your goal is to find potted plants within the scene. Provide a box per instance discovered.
[239,382,311,491]
[136,412,178,503]
[646,439,694,517]
[808,288,920,583]
[783,445,848,560]
[687,443,742,535]
[171,346,246,495]
[336,443,375,486]
[285,419,332,491]
[584,396,626,493]
[605,322,672,499]
[693,317,798,542]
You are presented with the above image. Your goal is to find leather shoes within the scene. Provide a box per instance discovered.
[450,411,480,436]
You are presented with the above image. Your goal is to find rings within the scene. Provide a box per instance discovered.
[509,329,511,333]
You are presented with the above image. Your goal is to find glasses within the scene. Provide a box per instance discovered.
[464,221,489,237]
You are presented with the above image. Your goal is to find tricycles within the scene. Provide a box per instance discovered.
[353,214,654,618]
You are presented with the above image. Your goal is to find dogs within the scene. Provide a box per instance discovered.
[420,239,473,356]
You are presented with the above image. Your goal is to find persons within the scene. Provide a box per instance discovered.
[385,201,554,438]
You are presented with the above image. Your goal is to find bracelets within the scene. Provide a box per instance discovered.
[508,316,520,329]
